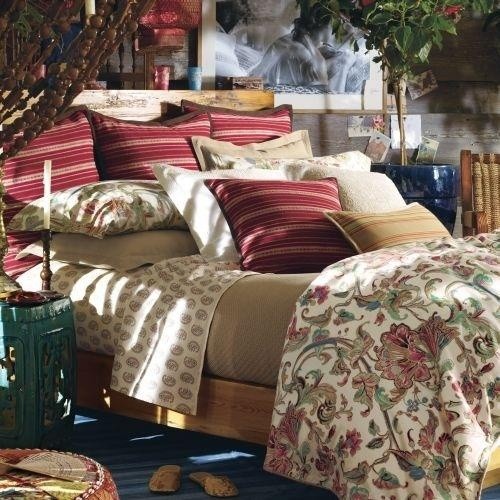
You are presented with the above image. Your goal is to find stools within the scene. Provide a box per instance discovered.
[0,299,78,449]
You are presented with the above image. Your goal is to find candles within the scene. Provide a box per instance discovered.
[43,160,53,230]
[85,0,95,15]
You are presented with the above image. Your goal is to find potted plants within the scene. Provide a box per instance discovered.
[298,0,500,238]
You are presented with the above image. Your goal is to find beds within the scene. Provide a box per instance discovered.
[0,89,500,500]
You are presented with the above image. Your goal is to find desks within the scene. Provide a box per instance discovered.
[0,448,121,500]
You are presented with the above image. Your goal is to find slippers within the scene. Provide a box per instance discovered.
[189,470,236,497]
[146,461,185,494]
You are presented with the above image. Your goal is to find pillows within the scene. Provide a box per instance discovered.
[8,177,185,238]
[180,100,293,143]
[212,151,371,175]
[15,233,196,274]
[199,174,349,272]
[318,199,456,255]
[83,104,214,176]
[154,158,285,270]
[190,129,314,172]
[7,103,101,234]
[304,167,405,214]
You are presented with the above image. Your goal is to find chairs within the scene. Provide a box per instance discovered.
[459,149,500,238]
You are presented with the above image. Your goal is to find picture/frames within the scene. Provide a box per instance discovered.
[195,0,386,115]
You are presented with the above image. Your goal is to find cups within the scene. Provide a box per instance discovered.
[187,67,202,90]
[153,65,170,90]
